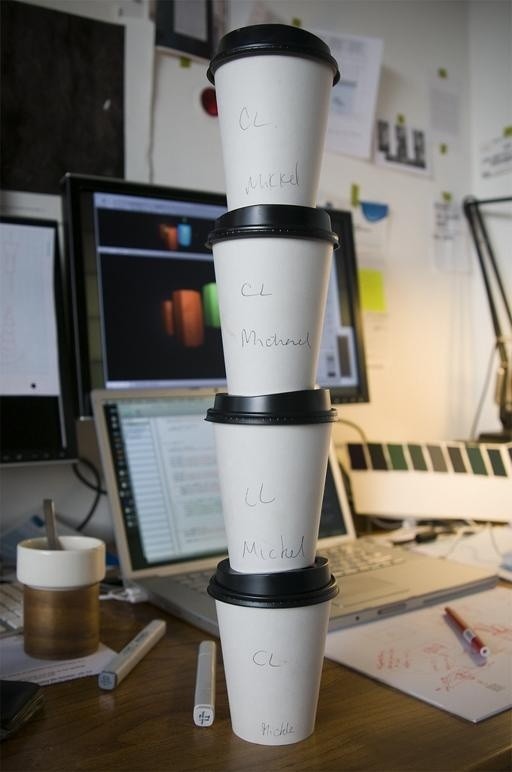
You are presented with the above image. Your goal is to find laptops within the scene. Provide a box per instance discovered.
[90,384,499,640]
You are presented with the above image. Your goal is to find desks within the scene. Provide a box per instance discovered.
[2,528,510,772]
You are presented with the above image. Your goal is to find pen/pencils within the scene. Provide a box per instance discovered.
[444,606,489,657]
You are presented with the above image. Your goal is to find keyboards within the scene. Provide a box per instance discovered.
[0,580,25,642]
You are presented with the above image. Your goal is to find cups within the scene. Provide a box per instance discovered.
[206,555,340,746]
[204,203,342,399]
[205,23,341,212]
[16,536,107,661]
[205,388,337,576]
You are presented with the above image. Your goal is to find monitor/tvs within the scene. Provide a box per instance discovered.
[0,213,80,469]
[60,173,369,423]
